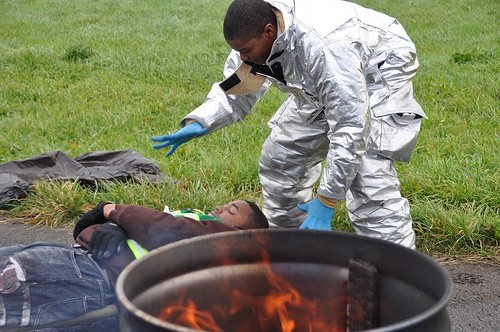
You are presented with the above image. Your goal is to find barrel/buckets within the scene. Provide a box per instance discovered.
[115,228,452,332]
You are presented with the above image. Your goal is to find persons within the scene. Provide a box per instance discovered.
[151,0,424,259]
[1,198,270,332]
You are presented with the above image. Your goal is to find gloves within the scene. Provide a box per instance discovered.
[297,194,339,231]
[88,219,128,260]
[150,119,209,157]
[73,201,114,240]
